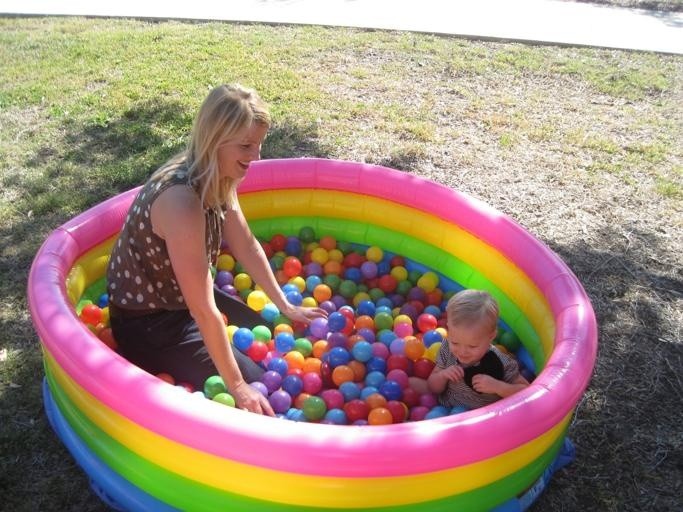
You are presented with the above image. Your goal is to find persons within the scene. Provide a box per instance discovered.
[426,289,531,410]
[105,80,329,420]
